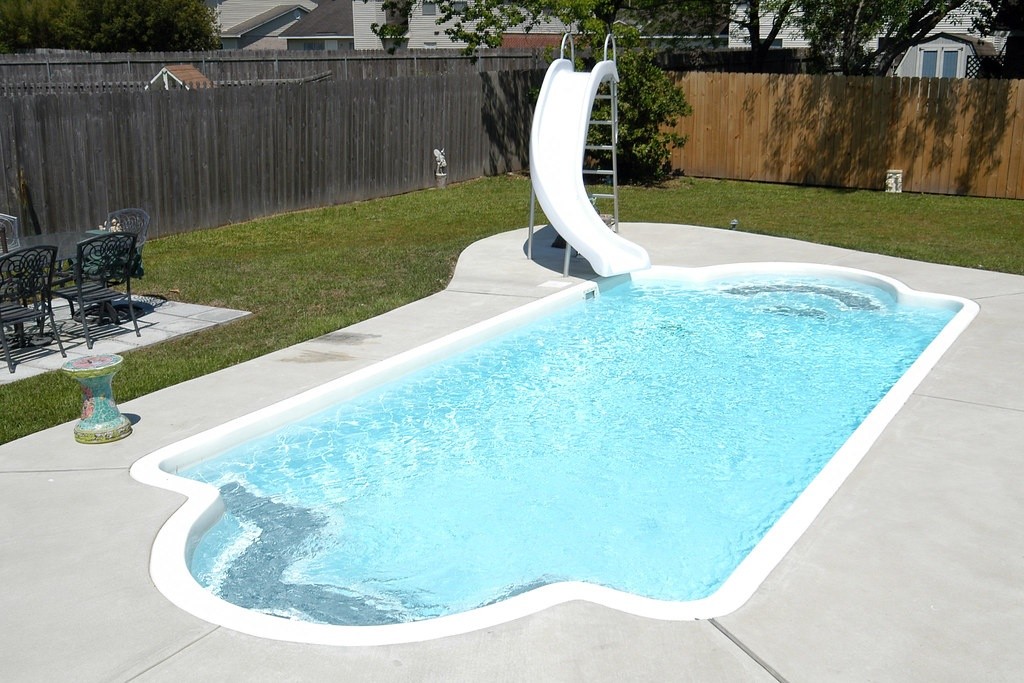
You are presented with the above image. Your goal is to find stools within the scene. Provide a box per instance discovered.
[60,352,132,445]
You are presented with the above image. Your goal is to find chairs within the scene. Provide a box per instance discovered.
[38,231,141,350]
[65,208,152,319]
[0,213,45,333]
[0,244,66,373]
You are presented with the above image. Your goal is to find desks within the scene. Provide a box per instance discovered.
[0,230,140,348]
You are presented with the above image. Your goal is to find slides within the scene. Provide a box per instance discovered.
[527,58,650,279]
